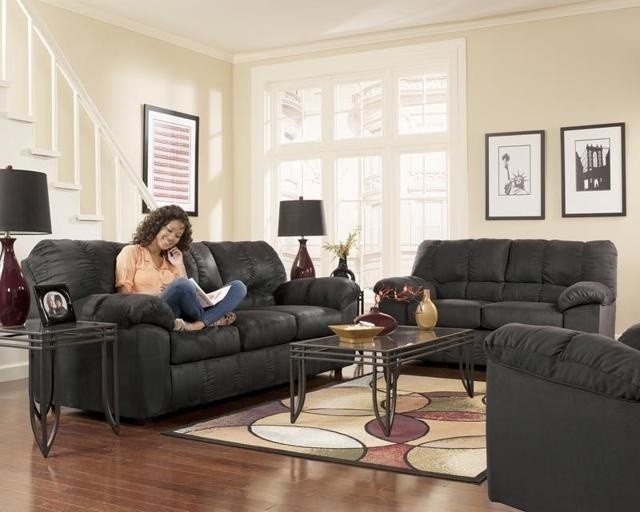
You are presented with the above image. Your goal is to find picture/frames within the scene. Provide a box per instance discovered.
[141,104,199,218]
[485,128,547,221]
[560,122,626,217]
[33,282,77,327]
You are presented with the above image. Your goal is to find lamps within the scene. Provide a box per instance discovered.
[278,198,326,279]
[1,169,52,329]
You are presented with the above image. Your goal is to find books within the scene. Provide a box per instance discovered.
[187,278,232,308]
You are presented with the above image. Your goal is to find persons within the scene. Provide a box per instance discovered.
[46,294,66,315]
[117,205,246,330]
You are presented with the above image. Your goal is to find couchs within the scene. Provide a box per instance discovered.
[484,322,638,511]
[373,237,618,368]
[19,239,360,422]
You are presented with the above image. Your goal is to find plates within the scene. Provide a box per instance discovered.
[328,324,385,344]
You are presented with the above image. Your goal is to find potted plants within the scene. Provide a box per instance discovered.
[324,230,357,280]
[351,284,423,338]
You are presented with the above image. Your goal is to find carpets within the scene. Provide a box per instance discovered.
[160,370,487,485]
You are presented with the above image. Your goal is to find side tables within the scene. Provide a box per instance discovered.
[1,320,120,457]
